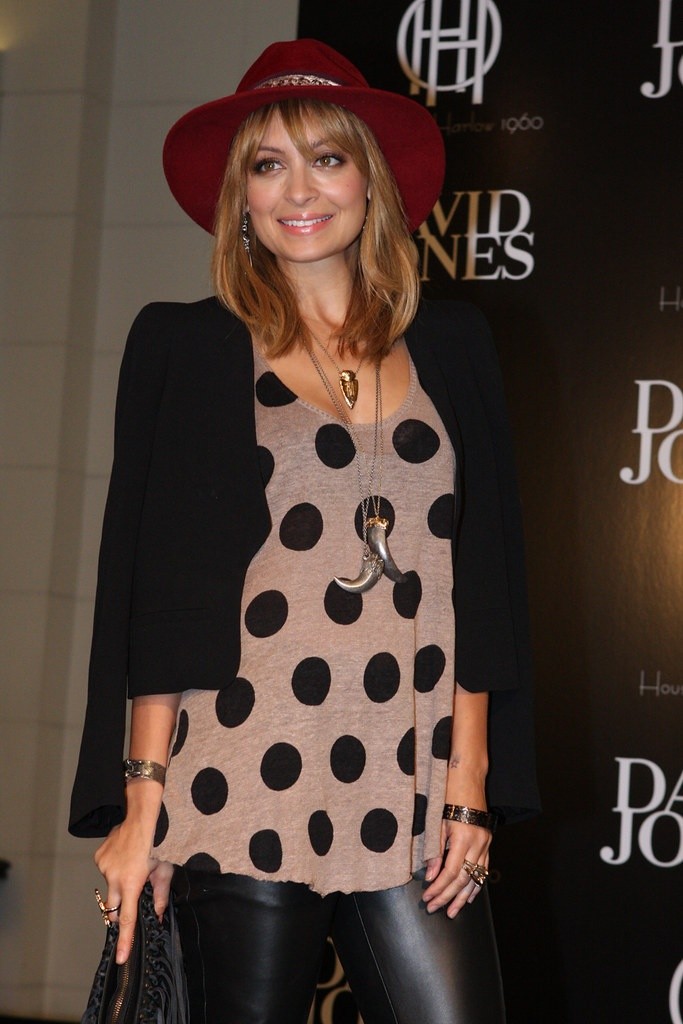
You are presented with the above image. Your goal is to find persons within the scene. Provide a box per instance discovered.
[93,37,509,1024]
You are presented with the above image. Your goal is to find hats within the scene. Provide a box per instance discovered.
[162,39,445,239]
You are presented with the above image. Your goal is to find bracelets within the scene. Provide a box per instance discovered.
[119,757,172,789]
[441,801,500,840]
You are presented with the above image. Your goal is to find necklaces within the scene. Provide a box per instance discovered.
[296,309,411,598]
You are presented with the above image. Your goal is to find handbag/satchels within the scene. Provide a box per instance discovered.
[82,881,191,1024]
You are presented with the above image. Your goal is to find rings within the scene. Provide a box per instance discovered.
[462,859,490,887]
[94,886,121,928]
